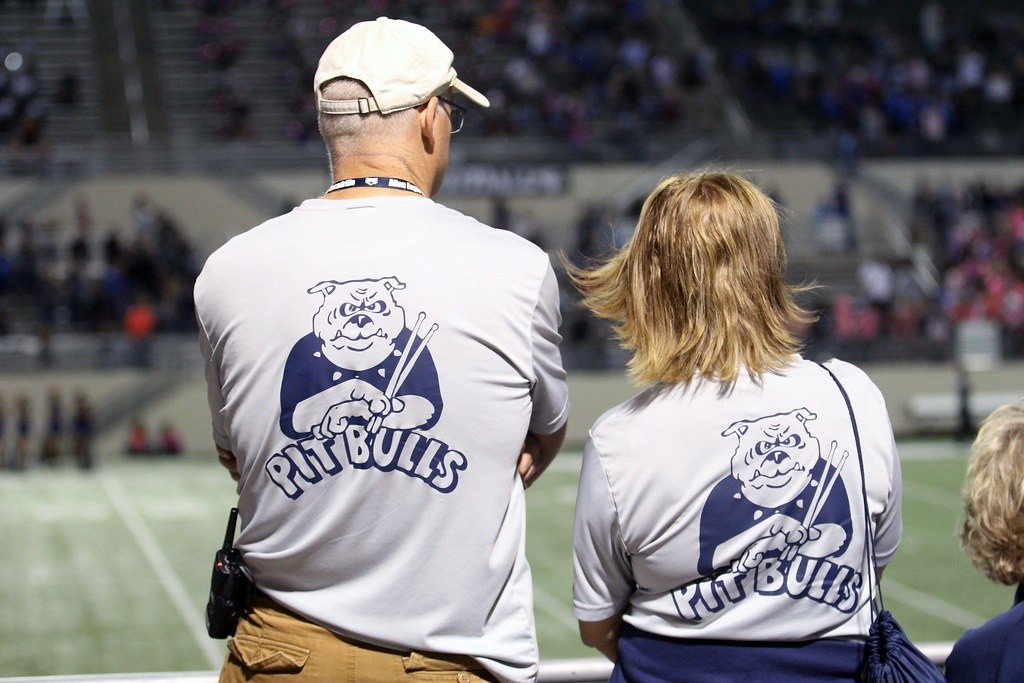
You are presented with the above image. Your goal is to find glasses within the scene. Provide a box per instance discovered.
[437,96,468,134]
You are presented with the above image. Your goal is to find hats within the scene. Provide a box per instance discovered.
[314,17,491,114]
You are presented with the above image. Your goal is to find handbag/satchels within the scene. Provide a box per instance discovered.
[861,611,946,683]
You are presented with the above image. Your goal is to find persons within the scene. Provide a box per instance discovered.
[40,393,62,466]
[11,393,29,469]
[72,400,96,470]
[188,16,571,683]
[941,403,1024,683]
[557,163,904,683]
[0,0,1023,372]
